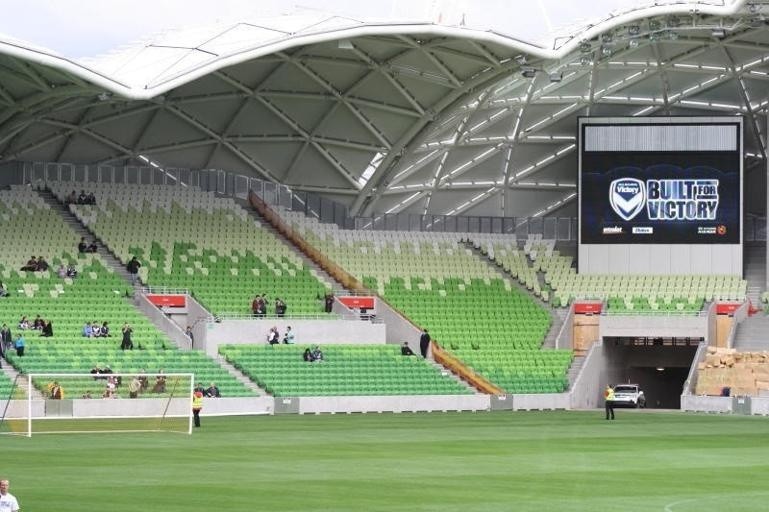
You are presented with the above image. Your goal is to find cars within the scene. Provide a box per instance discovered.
[611,384,646,408]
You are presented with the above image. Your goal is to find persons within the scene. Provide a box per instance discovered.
[0,479,20,512]
[253,294,323,362]
[402,329,430,359]
[47,365,221,400]
[192,388,203,427]
[0,315,133,358]
[185,326,193,349]
[604,384,616,419]
[0,237,140,297]
[65,190,96,205]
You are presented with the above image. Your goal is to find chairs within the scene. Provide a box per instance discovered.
[0,179,750,400]
